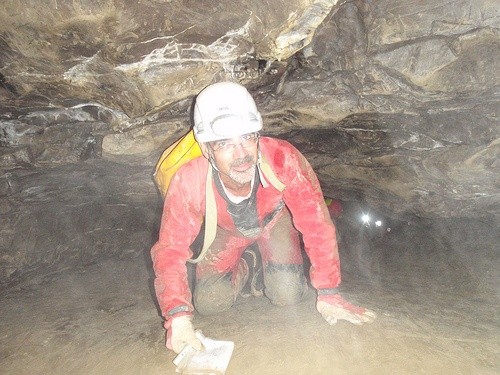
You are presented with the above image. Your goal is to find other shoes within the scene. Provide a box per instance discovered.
[241,249,265,298]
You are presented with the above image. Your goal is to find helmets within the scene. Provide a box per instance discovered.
[193,82,264,143]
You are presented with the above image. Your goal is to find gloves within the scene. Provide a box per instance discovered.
[164,318,203,355]
[316,293,378,326]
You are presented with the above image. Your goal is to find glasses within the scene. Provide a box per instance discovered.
[209,132,261,151]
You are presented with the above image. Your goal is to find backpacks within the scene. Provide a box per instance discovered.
[152,130,202,201]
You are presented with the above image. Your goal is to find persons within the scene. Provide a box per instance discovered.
[150,82,376,355]
